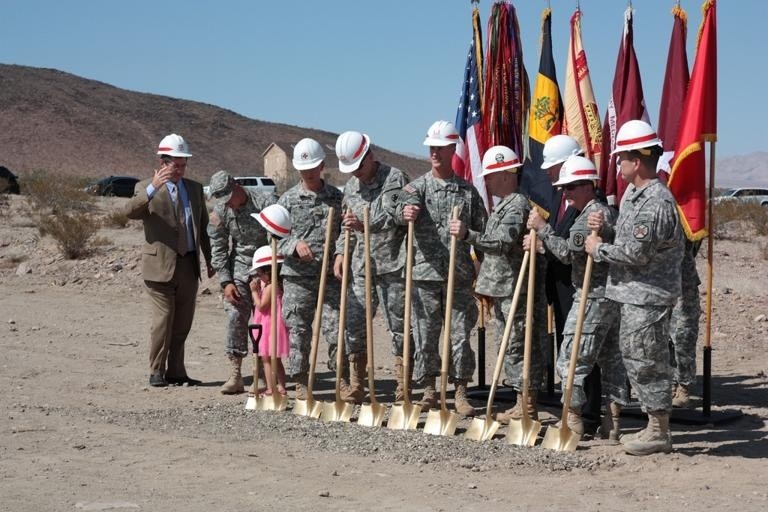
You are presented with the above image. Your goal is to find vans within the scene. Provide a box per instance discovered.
[706,188,768,208]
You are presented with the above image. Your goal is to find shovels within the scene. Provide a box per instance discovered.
[358,206,387,427]
[387,205,422,431]
[465,248,529,443]
[323,206,355,423]
[542,228,598,452]
[293,206,334,419]
[507,206,542,447]
[423,205,461,435]
[263,237,288,411]
[245,324,263,408]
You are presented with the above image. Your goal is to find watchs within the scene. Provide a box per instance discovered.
[538,246,545,254]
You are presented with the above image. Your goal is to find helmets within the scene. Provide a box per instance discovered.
[157,133,192,157]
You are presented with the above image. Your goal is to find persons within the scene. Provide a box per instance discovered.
[266,138,357,403]
[668,238,702,409]
[126,133,217,386]
[528,155,629,441]
[449,144,542,425]
[206,170,277,393]
[333,130,416,403]
[585,119,685,455]
[394,120,488,418]
[523,134,601,442]
[249,245,287,395]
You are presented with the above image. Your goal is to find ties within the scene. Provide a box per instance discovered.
[172,186,188,256]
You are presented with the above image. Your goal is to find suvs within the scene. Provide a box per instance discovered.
[201,177,276,199]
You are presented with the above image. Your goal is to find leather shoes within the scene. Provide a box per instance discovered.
[151,376,164,386]
[165,374,201,386]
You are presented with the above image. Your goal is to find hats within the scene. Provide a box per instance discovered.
[210,170,234,205]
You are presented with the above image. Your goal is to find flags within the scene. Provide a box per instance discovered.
[454,1,716,243]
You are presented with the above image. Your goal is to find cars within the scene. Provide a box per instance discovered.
[85,176,140,197]
